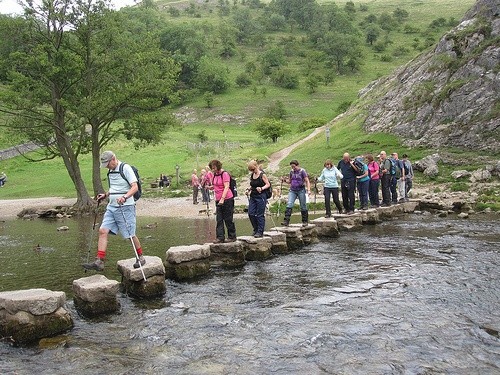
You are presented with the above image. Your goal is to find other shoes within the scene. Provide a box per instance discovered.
[82,260,105,271]
[213,238,223,244]
[344,198,406,215]
[324,213,331,218]
[254,233,264,238]
[226,238,237,243]
[133,254,146,269]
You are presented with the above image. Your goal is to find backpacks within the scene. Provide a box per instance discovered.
[403,163,409,175]
[212,170,238,197]
[257,170,272,198]
[107,162,141,200]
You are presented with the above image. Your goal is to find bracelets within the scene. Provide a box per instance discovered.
[261,187,262,191]
[123,196,126,202]
[105,193,106,197]
[308,190,310,191]
[222,197,224,199]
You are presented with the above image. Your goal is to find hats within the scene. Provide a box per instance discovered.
[100,150,114,169]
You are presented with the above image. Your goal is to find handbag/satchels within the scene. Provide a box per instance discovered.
[351,158,363,173]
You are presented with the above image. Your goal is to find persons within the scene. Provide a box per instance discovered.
[281,160,311,227]
[159,176,168,190]
[354,156,370,210]
[314,159,343,218]
[375,150,413,207]
[81,151,146,272]
[365,154,379,208]
[205,160,236,244]
[192,166,214,205]
[337,152,360,214]
[0,172,7,188]
[245,160,270,238]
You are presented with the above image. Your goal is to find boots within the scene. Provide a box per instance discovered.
[281,207,293,227]
[300,210,308,226]
